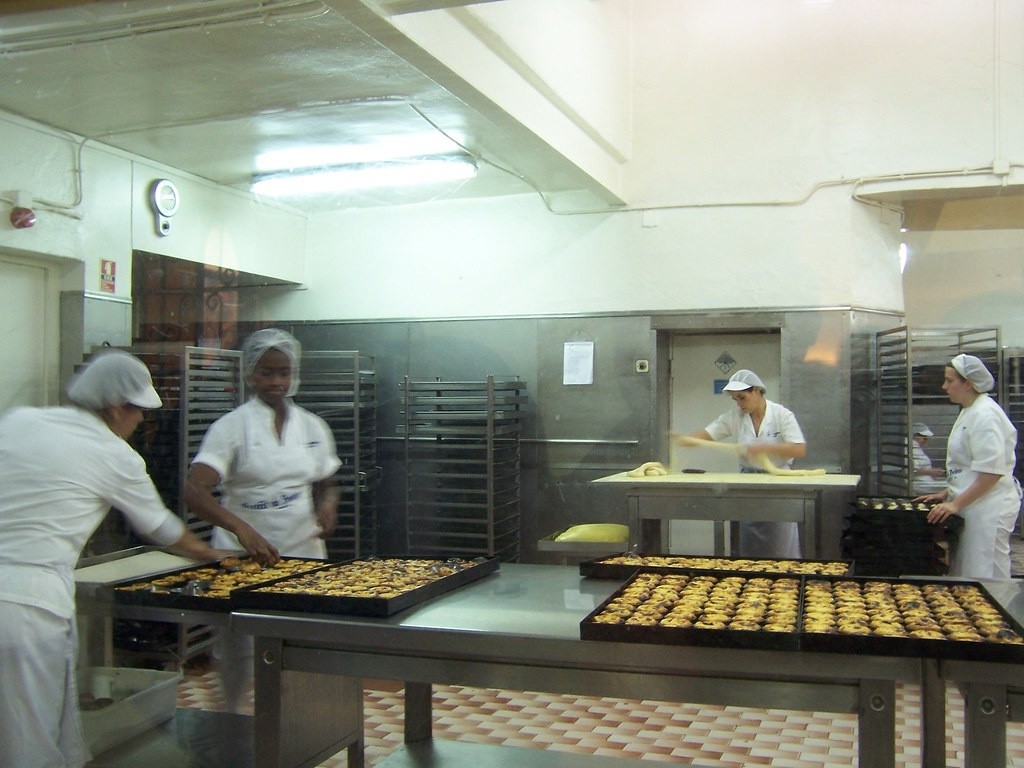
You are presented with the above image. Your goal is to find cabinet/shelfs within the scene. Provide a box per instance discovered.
[876,323,1024,499]
[74,340,530,768]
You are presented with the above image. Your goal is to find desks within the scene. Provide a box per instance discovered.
[628,490,819,561]
[229,561,1024,768]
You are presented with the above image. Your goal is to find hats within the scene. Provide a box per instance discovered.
[240,328,302,397]
[67,352,165,410]
[912,421,934,436]
[951,353,994,393]
[722,369,767,396]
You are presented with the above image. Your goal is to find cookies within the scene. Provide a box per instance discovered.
[804,578,1023,643]
[593,573,800,633]
[595,556,849,575]
[117,554,486,600]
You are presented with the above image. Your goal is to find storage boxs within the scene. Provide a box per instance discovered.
[74,665,182,755]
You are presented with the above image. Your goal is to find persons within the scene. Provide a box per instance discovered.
[912,353,1023,578]
[905,423,943,481]
[0,348,237,768]
[678,369,807,559]
[183,328,342,567]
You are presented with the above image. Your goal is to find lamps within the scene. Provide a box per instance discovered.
[249,154,478,199]
